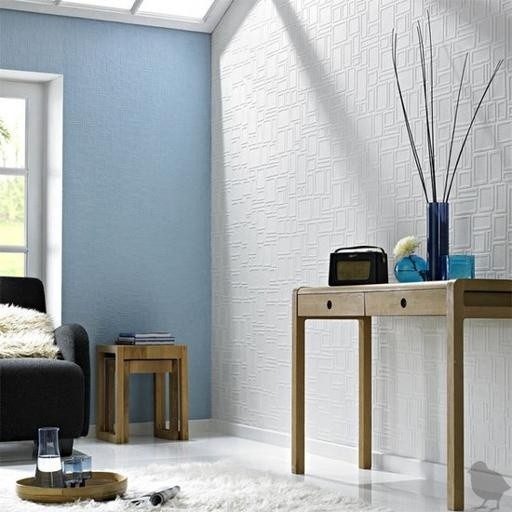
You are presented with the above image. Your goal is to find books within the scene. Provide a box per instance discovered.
[115,332,176,346]
[120,486,180,507]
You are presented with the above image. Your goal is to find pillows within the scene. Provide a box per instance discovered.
[0,303,59,360]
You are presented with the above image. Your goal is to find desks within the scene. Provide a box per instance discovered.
[291,279,512,511]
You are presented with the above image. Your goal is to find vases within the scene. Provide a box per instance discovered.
[393,255,427,282]
[427,202,449,280]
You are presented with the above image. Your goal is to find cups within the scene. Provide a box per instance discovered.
[64,459,82,486]
[74,454,93,478]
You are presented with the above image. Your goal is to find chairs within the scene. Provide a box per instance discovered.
[0,276,91,457]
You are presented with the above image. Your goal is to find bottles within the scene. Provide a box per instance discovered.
[36,428,64,486]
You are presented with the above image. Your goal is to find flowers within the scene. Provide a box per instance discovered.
[394,236,425,260]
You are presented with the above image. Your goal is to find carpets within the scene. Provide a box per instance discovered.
[0,460,391,511]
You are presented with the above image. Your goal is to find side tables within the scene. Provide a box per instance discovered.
[97,343,189,443]
